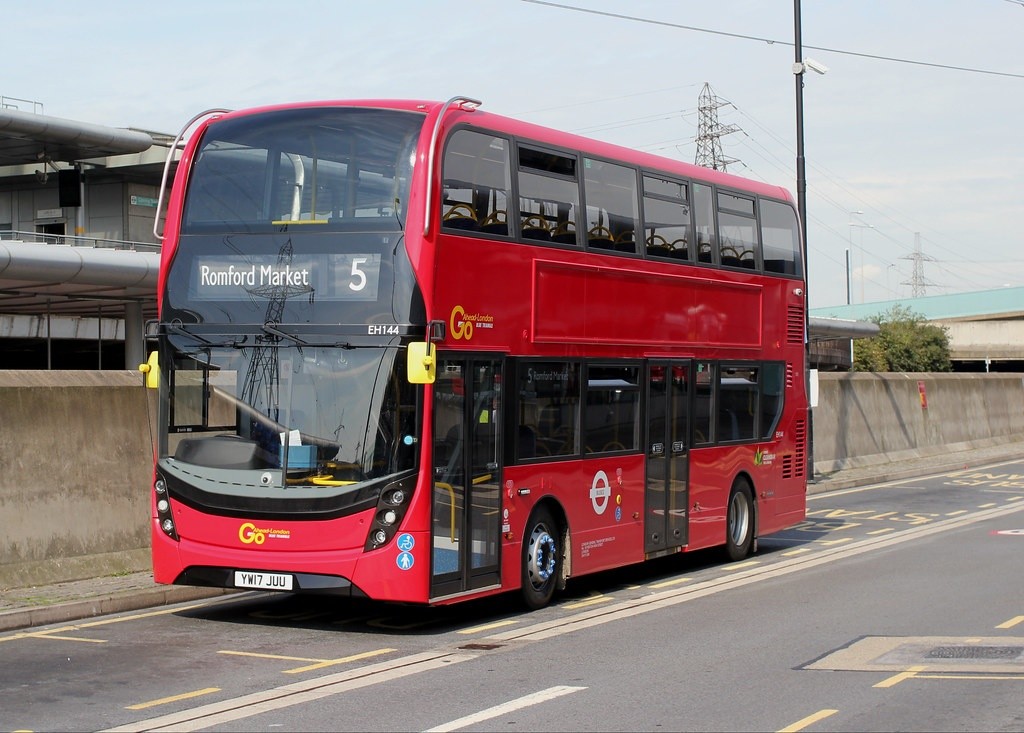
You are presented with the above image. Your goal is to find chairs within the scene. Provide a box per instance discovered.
[531,427,708,460]
[443,203,755,270]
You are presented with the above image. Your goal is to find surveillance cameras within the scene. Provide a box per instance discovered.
[803,57,829,74]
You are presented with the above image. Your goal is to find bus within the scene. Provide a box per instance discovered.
[137,96,810,610]
